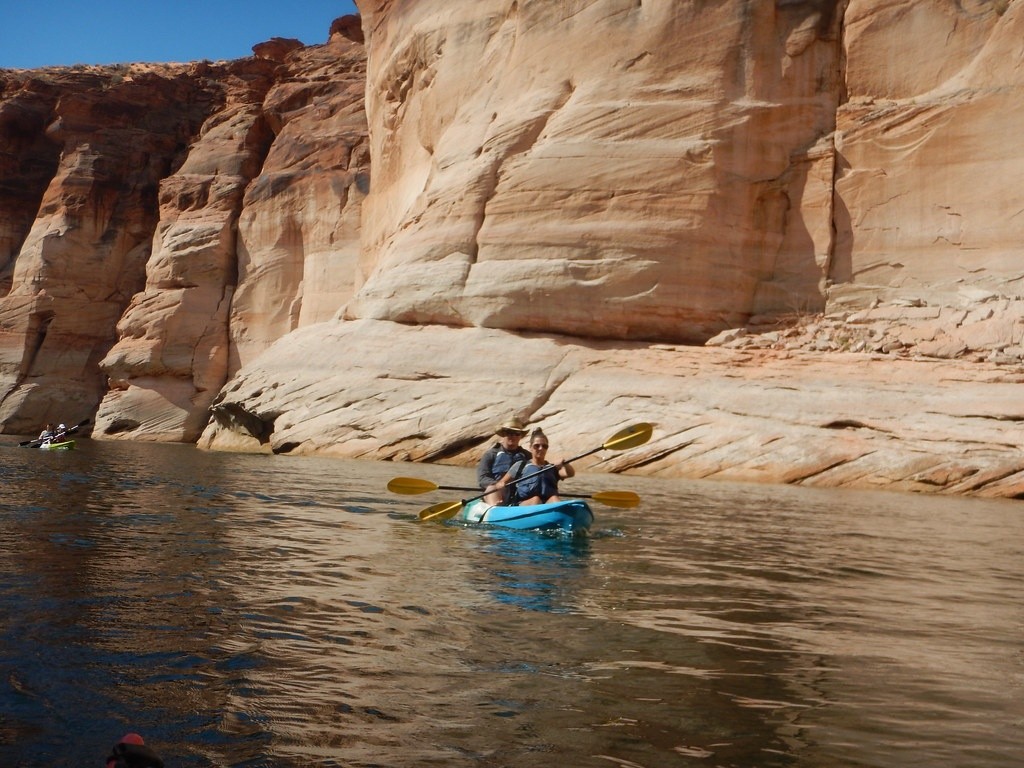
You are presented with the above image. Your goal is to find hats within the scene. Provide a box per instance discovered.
[59,423,65,429]
[495,416,530,438]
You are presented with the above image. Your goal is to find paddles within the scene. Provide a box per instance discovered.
[415,422,653,525]
[388,476,641,507]
[19,418,91,449]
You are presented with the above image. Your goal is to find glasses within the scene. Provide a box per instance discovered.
[506,431,522,436]
[533,444,549,449]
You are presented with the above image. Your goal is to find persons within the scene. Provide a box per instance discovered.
[496,428,575,506]
[477,415,532,505]
[38,422,66,443]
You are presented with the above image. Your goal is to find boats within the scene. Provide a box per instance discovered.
[37,439,76,450]
[459,493,595,545]
